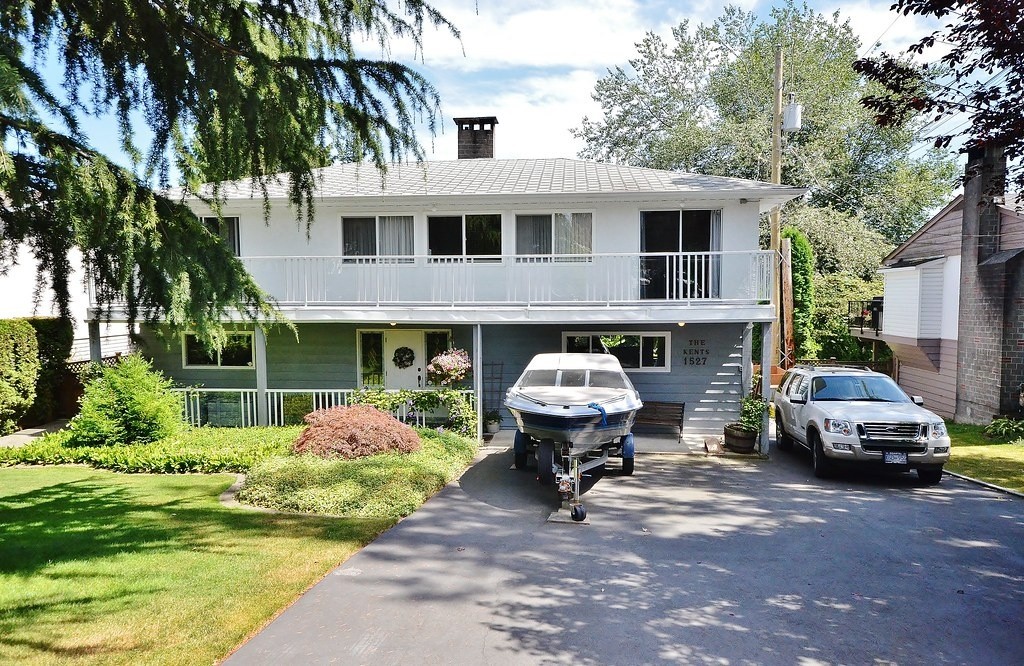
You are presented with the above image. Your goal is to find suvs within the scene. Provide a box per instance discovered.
[775,364,951,485]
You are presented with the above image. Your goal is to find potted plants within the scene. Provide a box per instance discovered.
[724,397,767,453]
[485,408,503,433]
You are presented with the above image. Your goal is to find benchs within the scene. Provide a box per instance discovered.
[634,401,685,443]
[56,352,122,419]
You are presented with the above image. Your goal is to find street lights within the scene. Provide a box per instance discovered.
[772,40,800,385]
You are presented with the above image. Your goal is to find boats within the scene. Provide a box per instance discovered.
[503,352,643,460]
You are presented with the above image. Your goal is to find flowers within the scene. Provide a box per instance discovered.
[427,348,472,387]
